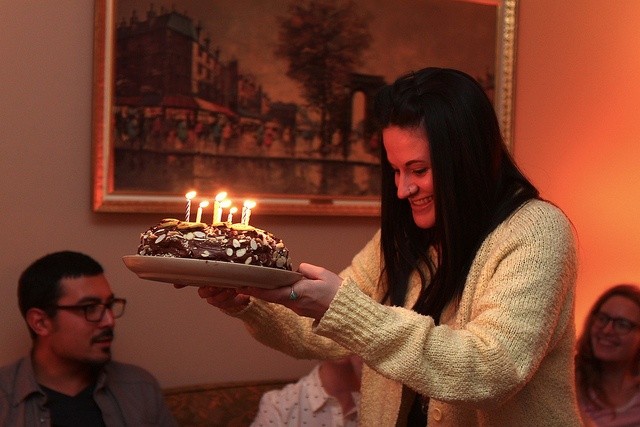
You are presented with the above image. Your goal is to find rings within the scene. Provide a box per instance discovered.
[289,286,300,301]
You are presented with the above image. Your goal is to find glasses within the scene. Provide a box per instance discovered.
[45,298,127,323]
[589,310,639,336]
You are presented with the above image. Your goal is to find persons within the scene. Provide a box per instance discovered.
[0,250,180,426]
[250,358,361,427]
[173,66,584,427]
[574,283,640,427]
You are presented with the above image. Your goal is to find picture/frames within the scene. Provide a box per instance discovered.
[93,0,516,215]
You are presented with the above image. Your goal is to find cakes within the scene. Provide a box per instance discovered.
[137,191,292,271]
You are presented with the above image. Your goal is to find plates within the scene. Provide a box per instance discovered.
[122,254,304,291]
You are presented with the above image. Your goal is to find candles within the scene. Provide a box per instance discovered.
[213,192,227,223]
[246,202,256,225]
[185,189,196,221]
[217,199,231,222]
[154,378,306,427]
[241,200,250,223]
[228,207,238,222]
[196,201,208,223]
